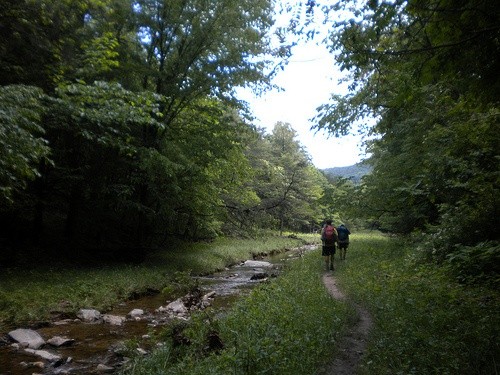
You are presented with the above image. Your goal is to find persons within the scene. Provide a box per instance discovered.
[320,220,339,272]
[336,224,351,261]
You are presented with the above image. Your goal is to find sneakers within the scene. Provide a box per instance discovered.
[330,265,334,270]
[326,266,329,272]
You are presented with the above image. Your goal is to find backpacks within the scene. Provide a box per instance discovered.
[337,226,348,241]
[323,225,336,243]
